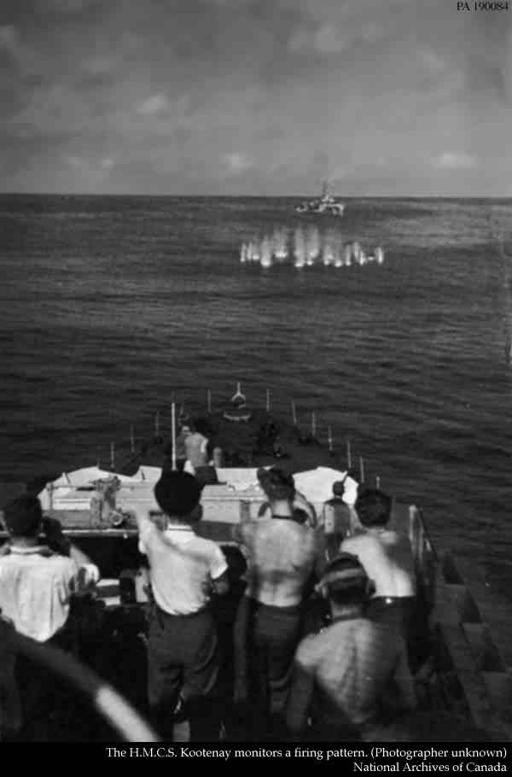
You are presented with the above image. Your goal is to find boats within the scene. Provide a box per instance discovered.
[1,377,509,743]
[297,183,346,217]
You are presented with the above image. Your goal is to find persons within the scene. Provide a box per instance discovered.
[340,489,430,709]
[287,552,421,742]
[231,466,327,744]
[174,422,192,472]
[320,481,352,561]
[207,446,226,469]
[1,490,101,743]
[184,418,220,491]
[131,466,231,743]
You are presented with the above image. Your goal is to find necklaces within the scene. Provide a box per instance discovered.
[332,611,366,623]
[270,515,294,520]
